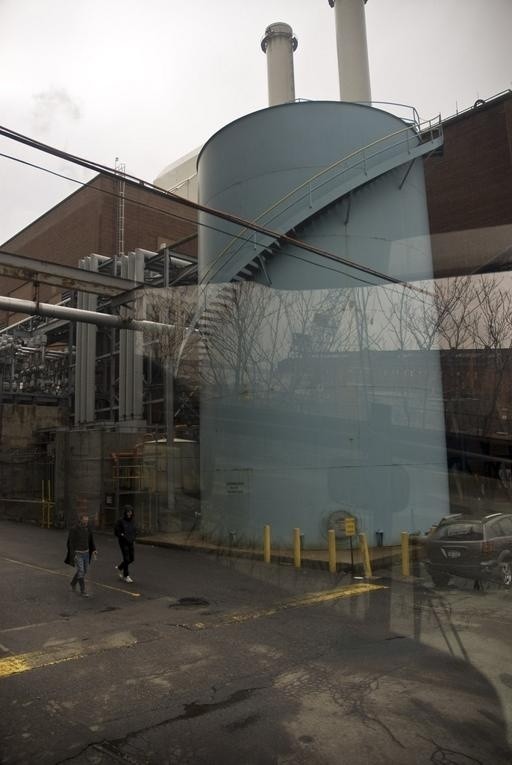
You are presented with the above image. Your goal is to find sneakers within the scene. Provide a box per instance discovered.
[123,576,133,583]
[70,581,79,591]
[81,592,88,598]
[115,566,122,578]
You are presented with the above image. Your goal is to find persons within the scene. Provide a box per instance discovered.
[114,504,139,583]
[65,513,98,597]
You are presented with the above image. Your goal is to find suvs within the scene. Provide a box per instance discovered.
[422,511,512,591]
